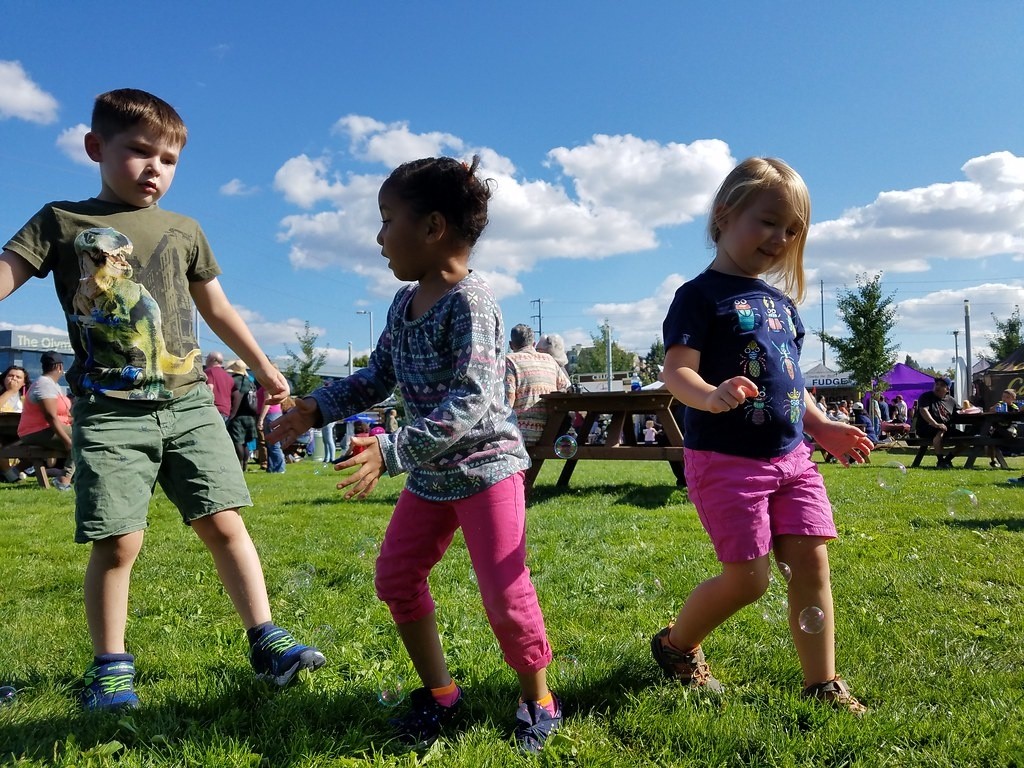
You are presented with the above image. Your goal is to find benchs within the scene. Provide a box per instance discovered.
[0,442,69,489]
[906,433,1024,446]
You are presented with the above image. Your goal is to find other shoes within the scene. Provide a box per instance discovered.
[261,462,267,470]
[936,459,954,468]
[52,478,72,490]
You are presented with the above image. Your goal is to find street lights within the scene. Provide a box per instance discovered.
[356,310,374,355]
[945,329,965,362]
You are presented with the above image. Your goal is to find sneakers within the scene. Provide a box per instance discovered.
[383,684,468,750]
[509,687,562,751]
[82,653,138,711]
[652,621,720,694]
[249,626,326,686]
[801,673,875,717]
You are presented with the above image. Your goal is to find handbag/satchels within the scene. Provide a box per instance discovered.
[248,381,257,411]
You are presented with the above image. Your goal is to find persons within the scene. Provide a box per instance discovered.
[341,421,372,460]
[323,421,338,466]
[812,394,918,463]
[203,351,316,473]
[0,350,76,491]
[649,155,875,718]
[266,155,561,756]
[0,88,324,718]
[910,375,1024,469]
[387,409,401,436]
[506,324,573,444]
[577,414,663,445]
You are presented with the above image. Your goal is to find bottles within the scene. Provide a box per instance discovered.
[995,403,1001,412]
[849,412,855,425]
[632,373,641,391]
[577,383,582,394]
[999,401,1007,412]
[1015,400,1024,412]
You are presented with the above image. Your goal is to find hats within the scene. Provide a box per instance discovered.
[41,350,64,364]
[224,360,248,376]
[852,402,864,410]
[934,376,952,388]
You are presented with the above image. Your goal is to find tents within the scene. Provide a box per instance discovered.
[970,344,1024,399]
[863,364,938,413]
[802,360,858,387]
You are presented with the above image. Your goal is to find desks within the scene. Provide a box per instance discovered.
[813,424,873,462]
[883,422,911,451]
[911,412,1024,469]
[526,390,684,506]
